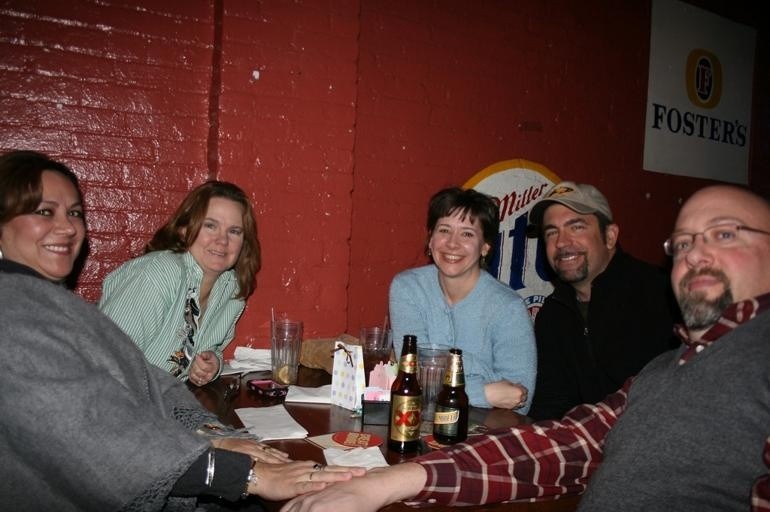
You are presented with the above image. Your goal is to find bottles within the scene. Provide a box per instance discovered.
[386,334,424,455]
[432,346,470,445]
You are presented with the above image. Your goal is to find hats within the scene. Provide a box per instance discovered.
[527,178,615,226]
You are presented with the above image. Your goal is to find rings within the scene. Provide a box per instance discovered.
[519,403,521,408]
[262,446,269,451]
[309,473,314,481]
[314,464,321,471]
[198,379,202,386]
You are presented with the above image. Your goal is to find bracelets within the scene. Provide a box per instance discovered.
[241,461,256,499]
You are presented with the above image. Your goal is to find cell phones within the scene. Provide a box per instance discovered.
[247,378,288,400]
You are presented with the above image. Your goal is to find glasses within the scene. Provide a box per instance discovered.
[661,223,769,259]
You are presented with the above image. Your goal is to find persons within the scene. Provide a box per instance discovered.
[280,185,770,512]
[0,150,367,512]
[537,183,679,426]
[390,187,538,417]
[96,181,260,390]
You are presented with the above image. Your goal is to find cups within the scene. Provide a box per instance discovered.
[358,326,393,388]
[414,344,452,423]
[270,319,304,386]
